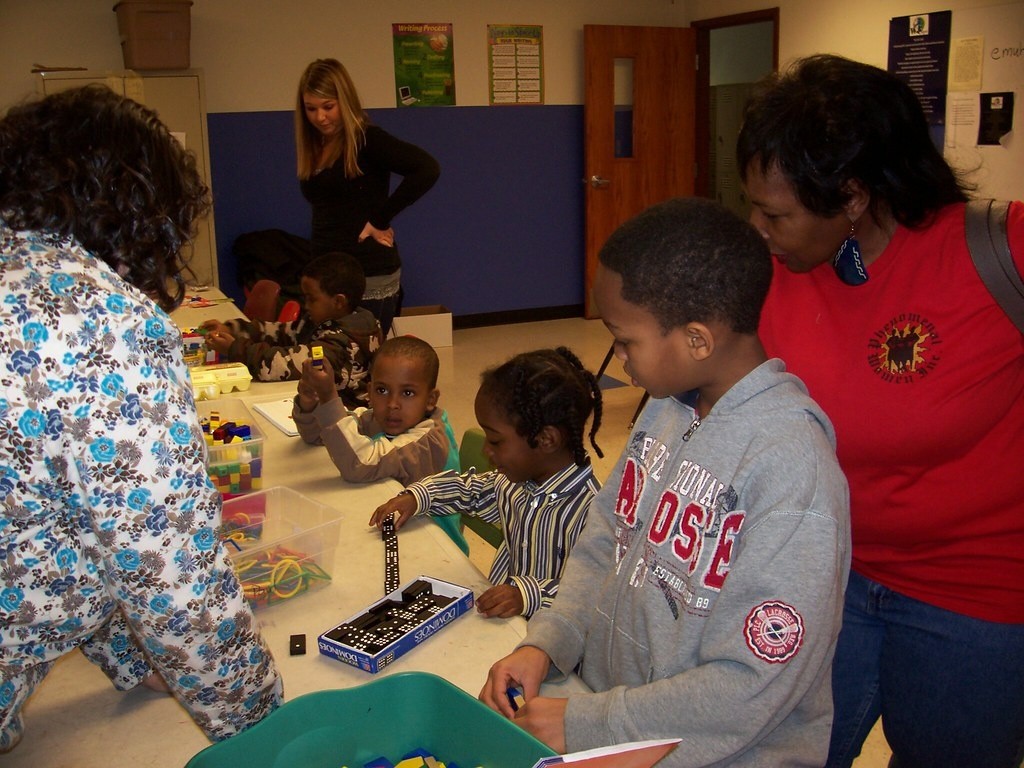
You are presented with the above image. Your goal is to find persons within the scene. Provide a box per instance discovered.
[291,333,475,558]
[735,53,1024,767]
[477,195,856,768]
[294,59,440,341]
[0,83,281,768]
[195,250,384,410]
[367,345,607,623]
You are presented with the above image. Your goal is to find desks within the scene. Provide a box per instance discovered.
[0,287,597,767]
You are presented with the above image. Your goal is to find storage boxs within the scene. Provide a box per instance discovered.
[212,485,342,618]
[390,306,454,349]
[111,0,193,73]
[184,670,562,768]
[189,398,266,508]
[316,574,475,674]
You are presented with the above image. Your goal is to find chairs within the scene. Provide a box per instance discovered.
[438,426,512,573]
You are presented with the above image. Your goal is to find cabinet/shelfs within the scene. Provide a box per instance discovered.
[32,66,221,290]
